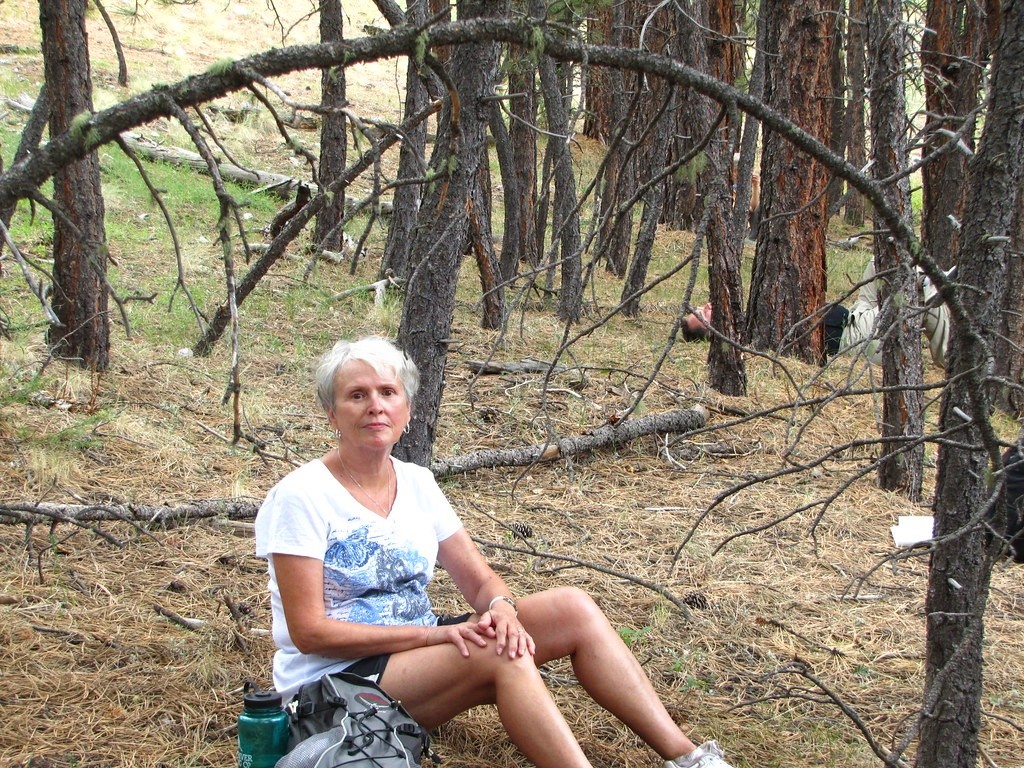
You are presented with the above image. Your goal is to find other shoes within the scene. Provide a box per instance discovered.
[662,740,734,768]
[924,266,956,307]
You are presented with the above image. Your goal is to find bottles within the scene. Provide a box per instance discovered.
[237,692,291,768]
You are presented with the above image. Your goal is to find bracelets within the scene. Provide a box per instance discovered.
[488,595,518,617]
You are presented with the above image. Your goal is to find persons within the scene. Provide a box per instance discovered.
[254,335,735,768]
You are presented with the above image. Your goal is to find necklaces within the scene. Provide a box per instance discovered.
[336,445,392,515]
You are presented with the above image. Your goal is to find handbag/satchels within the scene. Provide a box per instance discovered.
[274,672,441,768]
